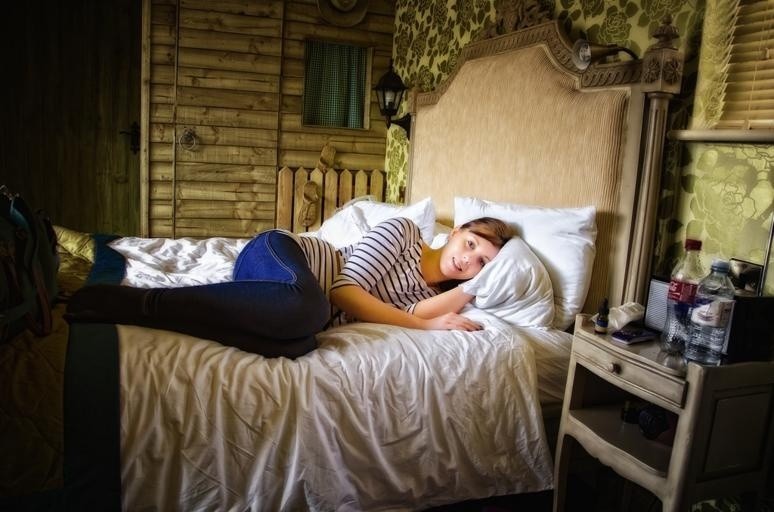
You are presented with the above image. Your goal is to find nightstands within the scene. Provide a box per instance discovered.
[550,313,773,512]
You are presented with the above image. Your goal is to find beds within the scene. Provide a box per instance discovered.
[2,21,687,512]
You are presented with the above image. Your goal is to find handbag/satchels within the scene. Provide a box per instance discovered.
[0,182,60,346]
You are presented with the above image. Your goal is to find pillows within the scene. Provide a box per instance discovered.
[326,195,597,333]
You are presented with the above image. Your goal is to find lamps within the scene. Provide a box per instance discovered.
[370,57,405,129]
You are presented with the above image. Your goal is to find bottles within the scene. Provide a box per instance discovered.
[659,239,707,354]
[684,259,735,366]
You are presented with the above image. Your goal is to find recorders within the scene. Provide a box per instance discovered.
[643,278,774,355]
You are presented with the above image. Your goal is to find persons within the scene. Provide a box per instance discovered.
[62,216,512,359]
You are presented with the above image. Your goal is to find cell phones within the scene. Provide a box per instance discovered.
[612,330,656,344]
